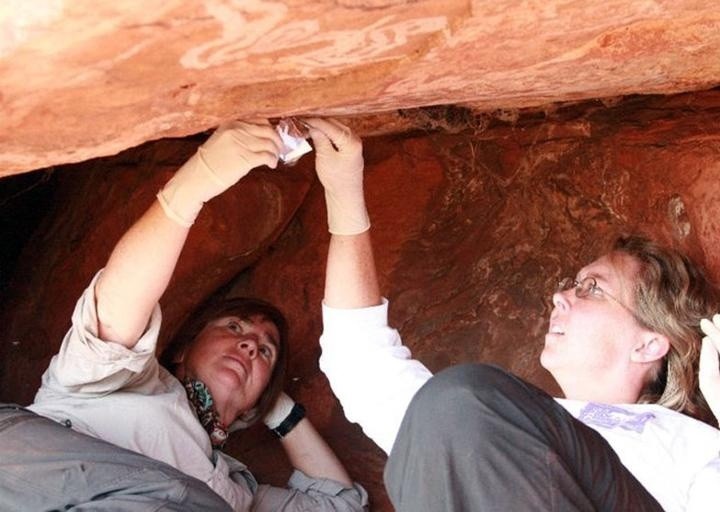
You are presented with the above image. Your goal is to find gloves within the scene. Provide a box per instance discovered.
[156,117,283,227]
[303,117,370,235]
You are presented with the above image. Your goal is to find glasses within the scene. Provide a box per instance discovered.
[557,275,626,311]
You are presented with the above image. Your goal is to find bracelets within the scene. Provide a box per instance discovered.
[269,401,306,440]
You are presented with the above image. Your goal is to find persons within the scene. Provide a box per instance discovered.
[303,117,719,512]
[0,117,371,511]
